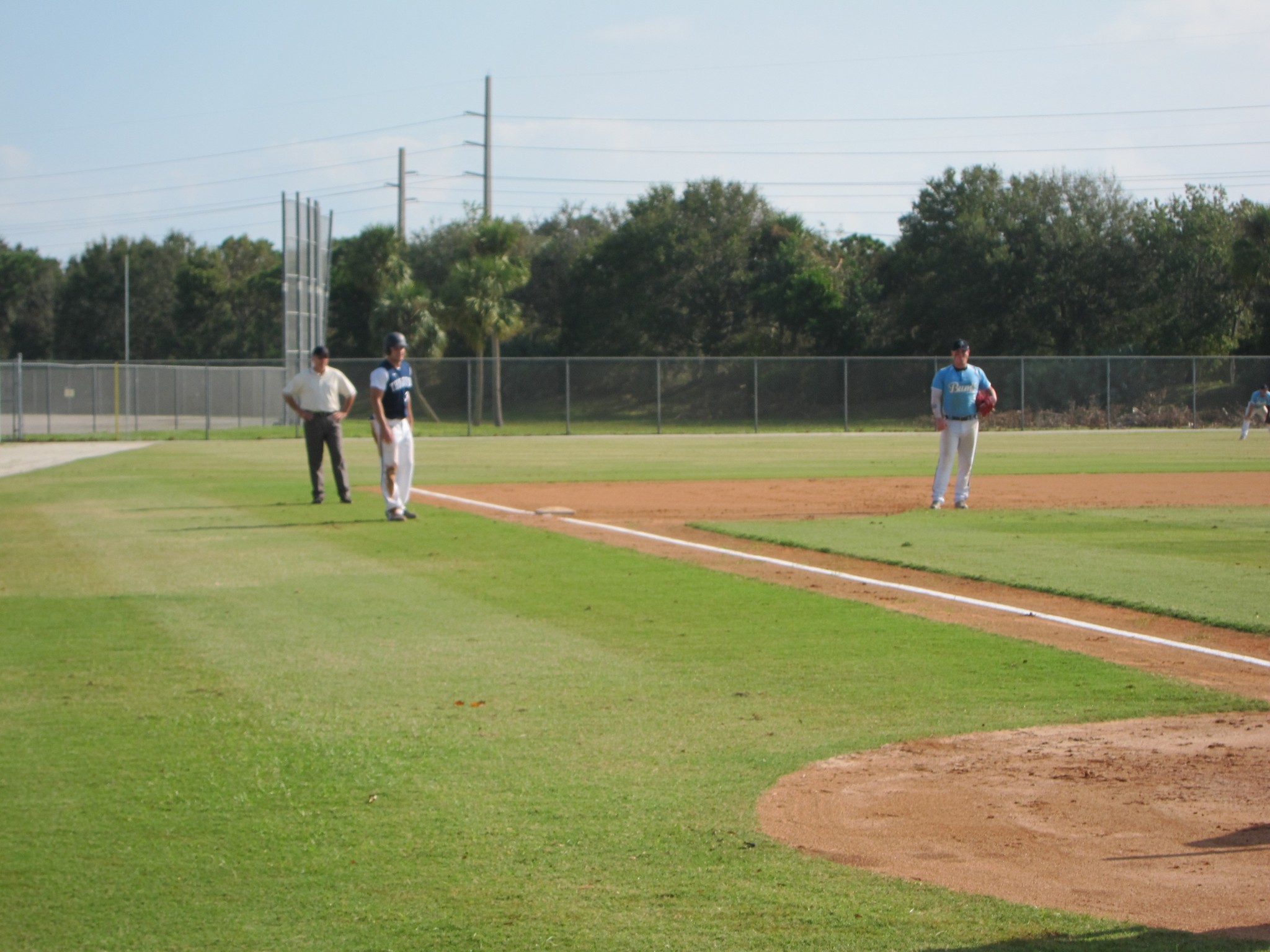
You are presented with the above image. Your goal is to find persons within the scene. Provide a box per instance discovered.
[1239,384,1270,440]
[281,346,358,504]
[369,331,419,522]
[929,338,998,510]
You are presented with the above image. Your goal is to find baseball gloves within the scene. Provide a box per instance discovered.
[975,388,994,417]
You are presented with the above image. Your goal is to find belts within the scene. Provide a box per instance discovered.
[943,415,978,420]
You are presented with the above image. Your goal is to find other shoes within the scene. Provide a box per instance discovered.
[1241,434,1246,440]
[957,500,967,509]
[341,497,352,503]
[930,501,941,509]
[313,497,323,503]
[385,507,405,521]
[403,509,418,519]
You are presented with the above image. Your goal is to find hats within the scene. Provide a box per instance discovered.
[386,333,408,350]
[951,338,969,351]
[314,346,328,356]
